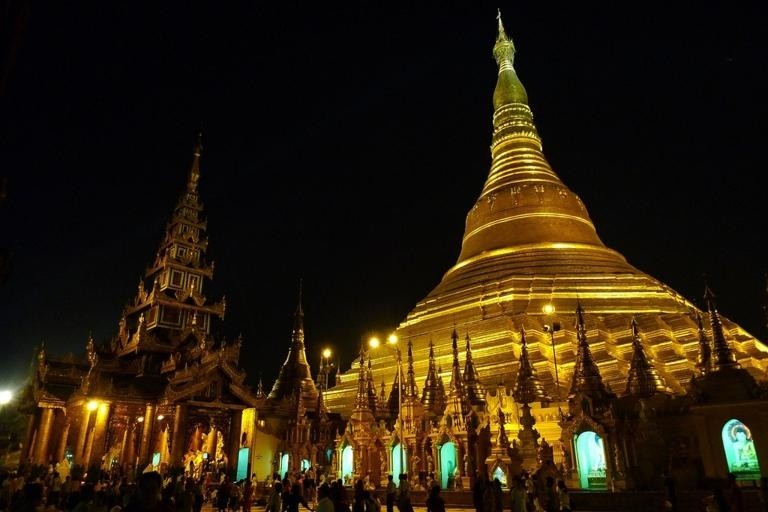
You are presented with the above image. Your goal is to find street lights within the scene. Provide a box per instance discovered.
[322,347,332,409]
[540,303,563,418]
[368,333,403,486]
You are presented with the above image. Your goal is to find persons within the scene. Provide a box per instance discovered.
[2,452,258,510]
[663,473,742,510]
[446,473,463,491]
[467,467,571,511]
[265,467,444,511]
